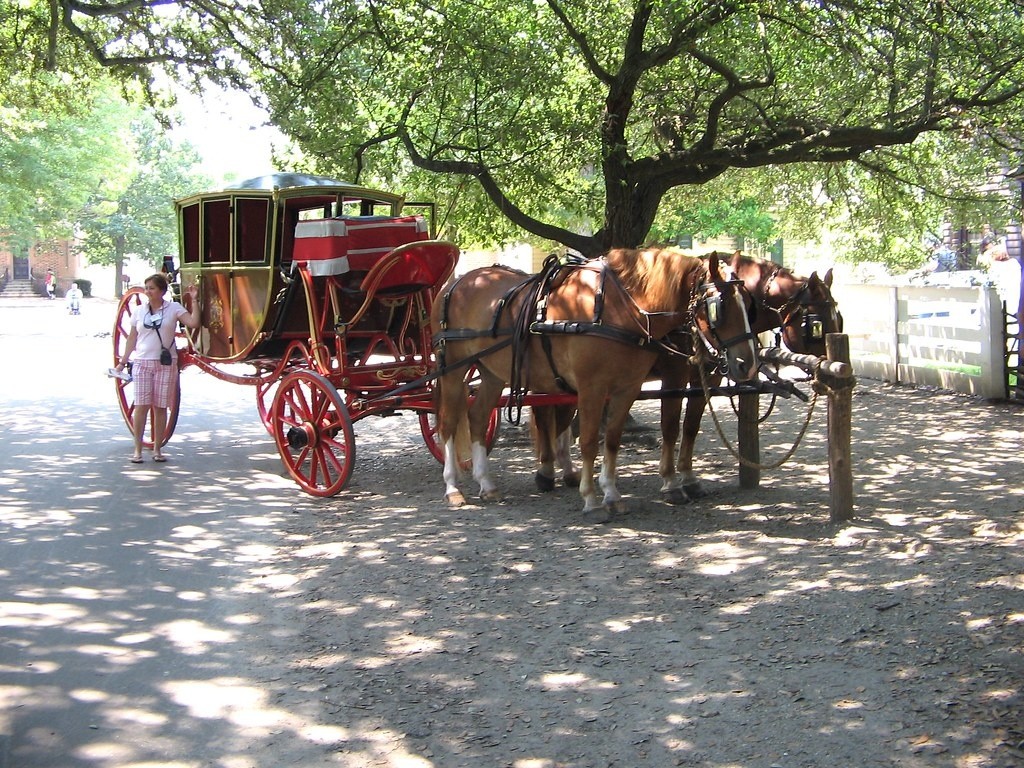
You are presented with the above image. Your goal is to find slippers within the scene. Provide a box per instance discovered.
[152,455,166,462]
[131,456,143,463]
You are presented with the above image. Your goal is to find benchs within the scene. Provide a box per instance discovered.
[289,213,430,279]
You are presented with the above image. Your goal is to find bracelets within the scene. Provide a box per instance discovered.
[192,300,198,302]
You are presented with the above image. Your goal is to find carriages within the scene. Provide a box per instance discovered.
[111,171,858,525]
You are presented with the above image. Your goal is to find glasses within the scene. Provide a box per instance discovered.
[143,311,163,329]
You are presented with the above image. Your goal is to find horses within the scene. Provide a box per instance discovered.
[530,252,844,523]
[432,248,756,524]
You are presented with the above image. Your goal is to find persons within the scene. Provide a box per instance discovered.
[115,274,201,463]
[44,268,57,299]
[915,232,1022,367]
[66,283,83,309]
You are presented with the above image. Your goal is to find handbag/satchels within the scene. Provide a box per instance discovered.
[47,284,54,292]
